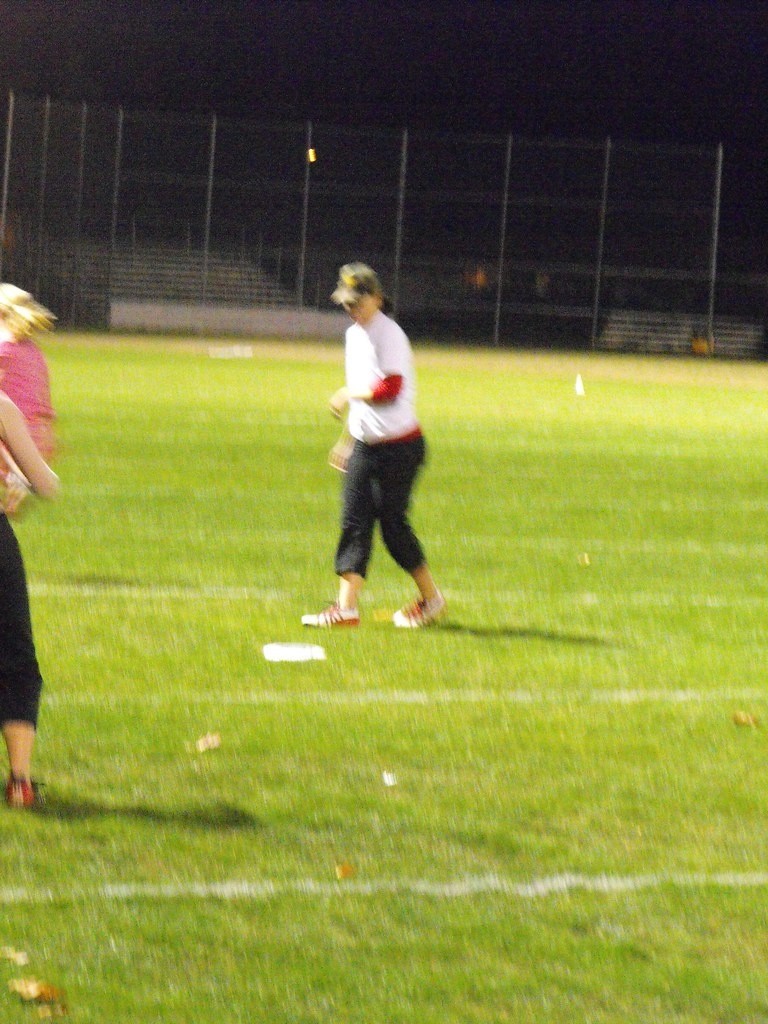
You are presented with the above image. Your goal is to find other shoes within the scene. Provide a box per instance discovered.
[5,771,44,809]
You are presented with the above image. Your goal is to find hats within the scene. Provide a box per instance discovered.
[329,261,379,305]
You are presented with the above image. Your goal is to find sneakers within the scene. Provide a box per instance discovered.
[302,601,360,627]
[394,592,446,628]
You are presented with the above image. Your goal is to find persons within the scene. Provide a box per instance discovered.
[299,261,449,633]
[0,391,60,812]
[0,282,58,493]
